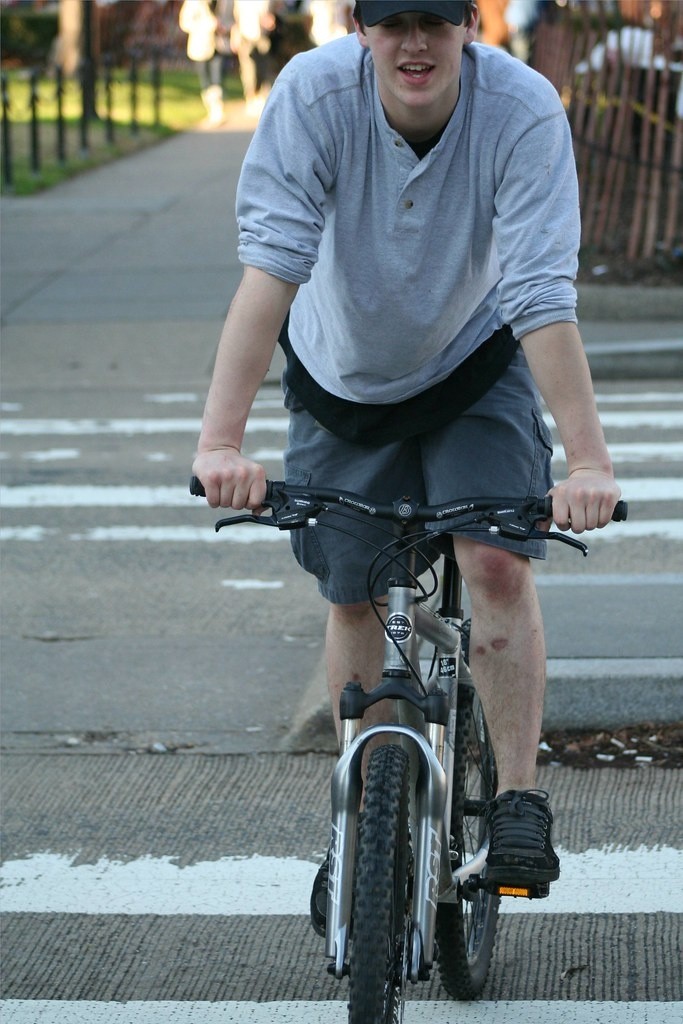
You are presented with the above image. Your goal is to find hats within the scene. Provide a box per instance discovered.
[359,0,466,27]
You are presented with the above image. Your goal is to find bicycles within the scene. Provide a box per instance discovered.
[190,466,627,1023]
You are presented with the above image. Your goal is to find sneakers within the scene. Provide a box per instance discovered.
[310,848,328,937]
[481,788,561,884]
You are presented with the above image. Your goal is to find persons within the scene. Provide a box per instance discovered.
[173,0,621,888]
